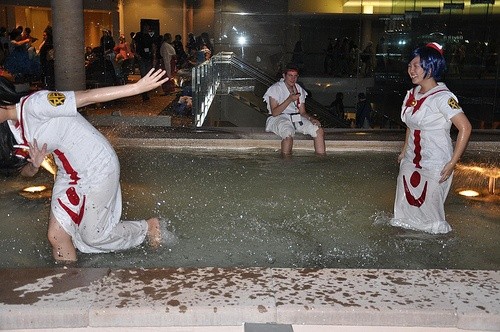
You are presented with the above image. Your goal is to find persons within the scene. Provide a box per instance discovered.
[324,36,356,77]
[84,29,134,87]
[0,68,169,262]
[355,93,372,129]
[291,41,304,77]
[393,46,471,223]
[361,36,399,74]
[129,24,213,111]
[330,92,344,119]
[0,25,56,89]
[262,66,326,156]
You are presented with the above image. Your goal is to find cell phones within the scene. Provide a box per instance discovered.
[295,93,301,96]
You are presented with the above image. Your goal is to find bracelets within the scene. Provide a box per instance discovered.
[308,116,311,121]
[449,161,456,170]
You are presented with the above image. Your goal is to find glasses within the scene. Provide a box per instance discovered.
[285,73,299,78]
